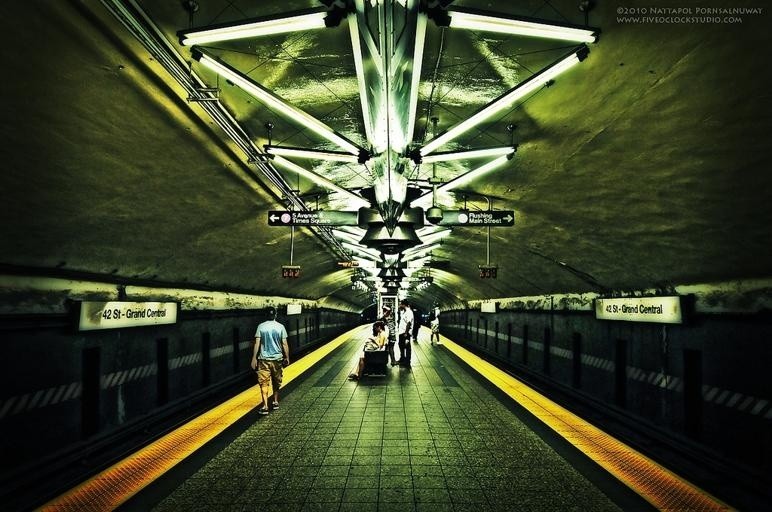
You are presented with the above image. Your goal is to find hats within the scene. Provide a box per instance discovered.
[266,307,276,315]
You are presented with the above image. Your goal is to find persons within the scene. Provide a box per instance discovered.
[397,299,414,366]
[429,302,443,345]
[251,306,290,414]
[347,321,385,381]
[379,304,399,366]
[413,306,422,342]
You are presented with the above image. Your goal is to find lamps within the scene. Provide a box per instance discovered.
[175,2,602,295]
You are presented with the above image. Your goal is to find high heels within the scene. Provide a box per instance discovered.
[348,374,360,380]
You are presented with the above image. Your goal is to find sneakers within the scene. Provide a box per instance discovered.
[258,408,269,415]
[272,401,279,409]
